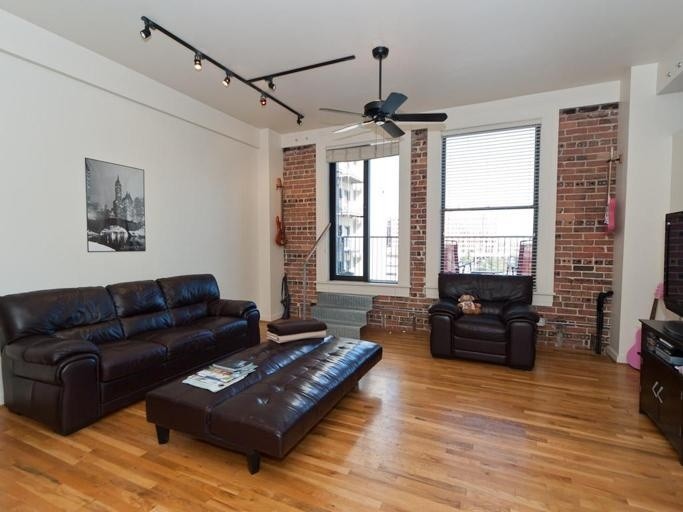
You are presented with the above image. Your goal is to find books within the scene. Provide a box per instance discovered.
[181,358,258,393]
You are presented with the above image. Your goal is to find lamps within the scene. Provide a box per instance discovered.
[138,15,302,129]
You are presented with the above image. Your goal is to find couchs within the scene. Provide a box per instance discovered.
[0,273,261,438]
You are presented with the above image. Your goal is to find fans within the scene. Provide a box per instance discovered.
[317,46,448,139]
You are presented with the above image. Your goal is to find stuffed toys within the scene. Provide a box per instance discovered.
[456,294,481,314]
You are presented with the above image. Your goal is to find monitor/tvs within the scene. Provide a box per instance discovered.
[664,210,683,342]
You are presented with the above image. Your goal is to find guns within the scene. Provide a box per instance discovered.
[281,273,290,319]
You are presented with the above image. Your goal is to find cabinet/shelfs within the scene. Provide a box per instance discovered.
[635,316,683,466]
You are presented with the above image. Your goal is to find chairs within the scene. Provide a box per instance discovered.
[427,271,541,372]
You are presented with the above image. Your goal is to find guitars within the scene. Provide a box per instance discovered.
[276,178,288,246]
[627,283,664,370]
